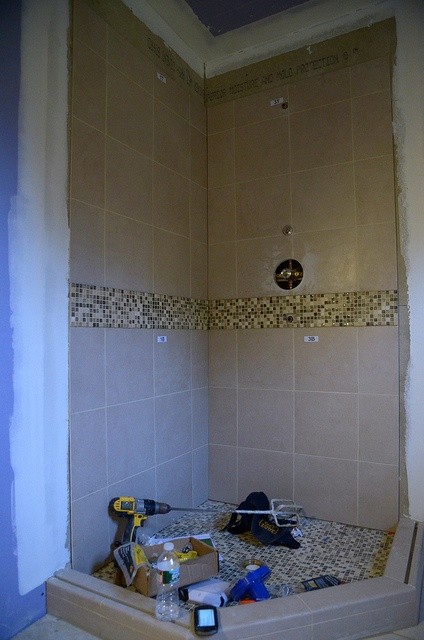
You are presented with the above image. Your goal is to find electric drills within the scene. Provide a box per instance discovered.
[107,495,302,547]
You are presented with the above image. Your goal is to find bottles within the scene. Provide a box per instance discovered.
[156,542,180,623]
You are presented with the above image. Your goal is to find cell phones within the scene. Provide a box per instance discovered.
[193,604,219,637]
